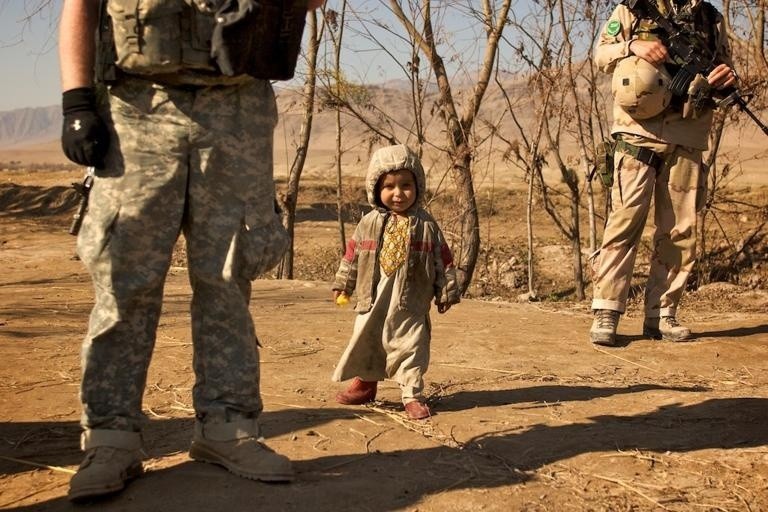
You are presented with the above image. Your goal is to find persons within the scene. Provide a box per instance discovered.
[60,1,302,501]
[588,0,739,346]
[330,144,458,418]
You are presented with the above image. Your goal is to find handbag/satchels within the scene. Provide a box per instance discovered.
[595,140,616,187]
[107,0,309,81]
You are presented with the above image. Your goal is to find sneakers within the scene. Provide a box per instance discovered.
[590,310,620,345]
[68,431,144,500]
[189,421,294,481]
[643,318,691,342]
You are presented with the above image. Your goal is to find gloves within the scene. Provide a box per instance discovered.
[62,86,108,169]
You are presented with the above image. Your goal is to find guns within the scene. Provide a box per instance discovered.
[617,0,768,138]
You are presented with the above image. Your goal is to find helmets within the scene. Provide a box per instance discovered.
[610,57,673,121]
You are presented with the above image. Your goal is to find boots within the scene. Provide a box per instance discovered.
[335,377,377,405]
[405,402,430,418]
